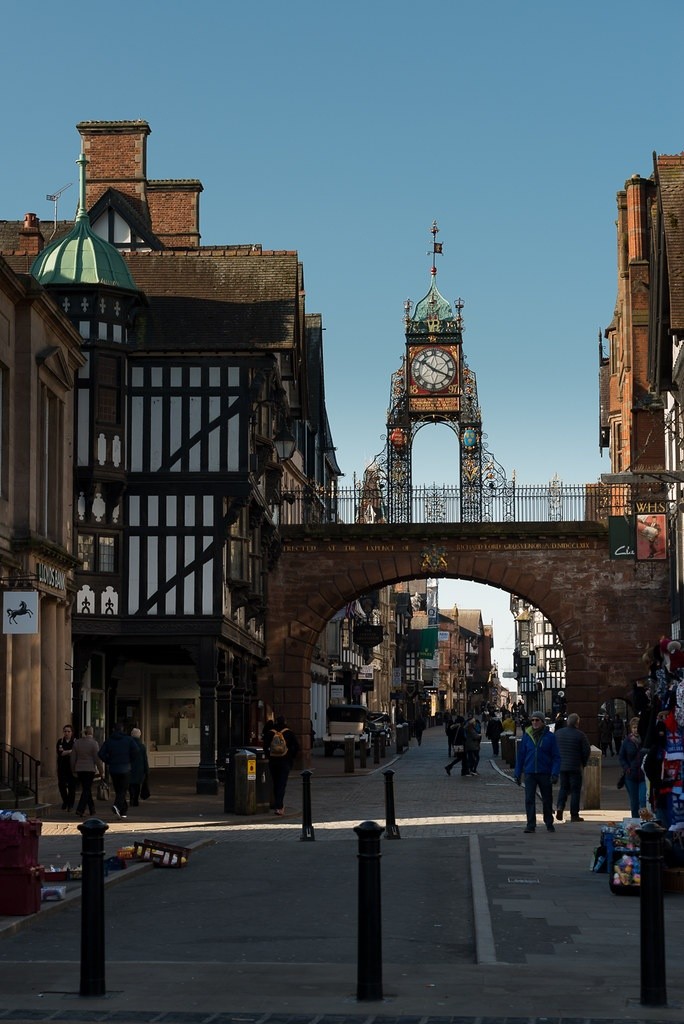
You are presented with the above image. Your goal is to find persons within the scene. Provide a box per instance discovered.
[382,714,391,723]
[481,702,567,757]
[56,724,104,817]
[514,712,560,834]
[617,718,646,817]
[598,713,628,757]
[444,709,482,775]
[97,723,140,817]
[260,715,298,815]
[128,728,148,808]
[413,714,426,745]
[397,712,406,725]
[554,713,591,822]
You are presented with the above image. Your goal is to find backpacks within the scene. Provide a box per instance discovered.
[269,728,290,756]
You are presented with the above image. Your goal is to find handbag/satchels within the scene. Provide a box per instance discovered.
[454,745,464,752]
[617,771,626,788]
[97,778,109,801]
[139,779,150,800]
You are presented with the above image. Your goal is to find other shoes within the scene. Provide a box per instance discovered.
[75,811,84,816]
[571,816,584,822]
[61,803,67,810]
[122,815,127,817]
[445,765,478,777]
[90,811,96,815]
[524,827,536,833]
[547,824,555,832]
[556,805,562,820]
[67,808,72,812]
[274,809,284,815]
[112,806,120,818]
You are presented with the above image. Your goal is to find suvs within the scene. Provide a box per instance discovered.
[322,703,393,758]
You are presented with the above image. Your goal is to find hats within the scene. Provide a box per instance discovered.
[531,711,545,722]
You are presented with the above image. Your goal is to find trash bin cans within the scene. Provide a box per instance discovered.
[500,731,516,759]
[223,746,274,815]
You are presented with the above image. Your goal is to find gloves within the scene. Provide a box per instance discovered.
[550,774,558,784]
[516,775,521,786]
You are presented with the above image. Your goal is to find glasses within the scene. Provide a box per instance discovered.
[531,718,540,722]
